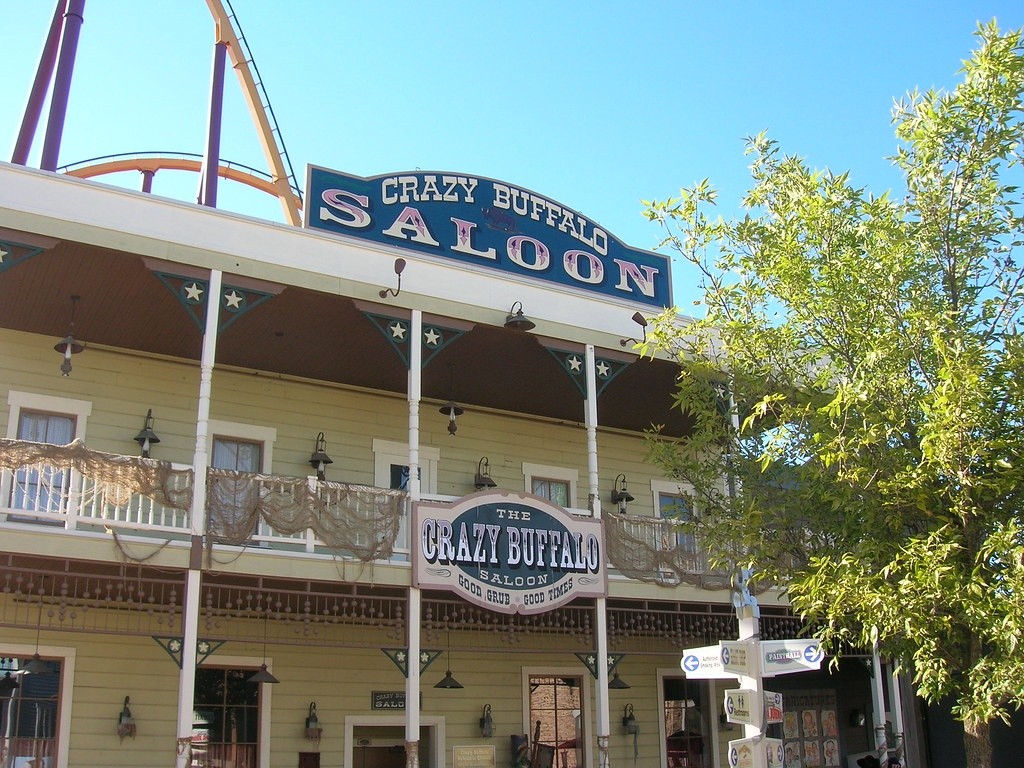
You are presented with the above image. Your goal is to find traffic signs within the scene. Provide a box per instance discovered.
[681,638,824,768]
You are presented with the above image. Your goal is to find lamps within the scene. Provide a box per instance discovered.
[503,300,536,333]
[479,704,496,737]
[378,257,406,298]
[308,431,333,481]
[54,294,84,377]
[438,362,464,436]
[246,614,280,684]
[118,696,135,735]
[611,472,635,514]
[608,654,631,689]
[622,703,640,735]
[306,703,322,739]
[15,594,52,674]
[432,624,463,689]
[474,456,497,491]
[620,311,649,348]
[133,408,161,458]
[720,703,734,730]
